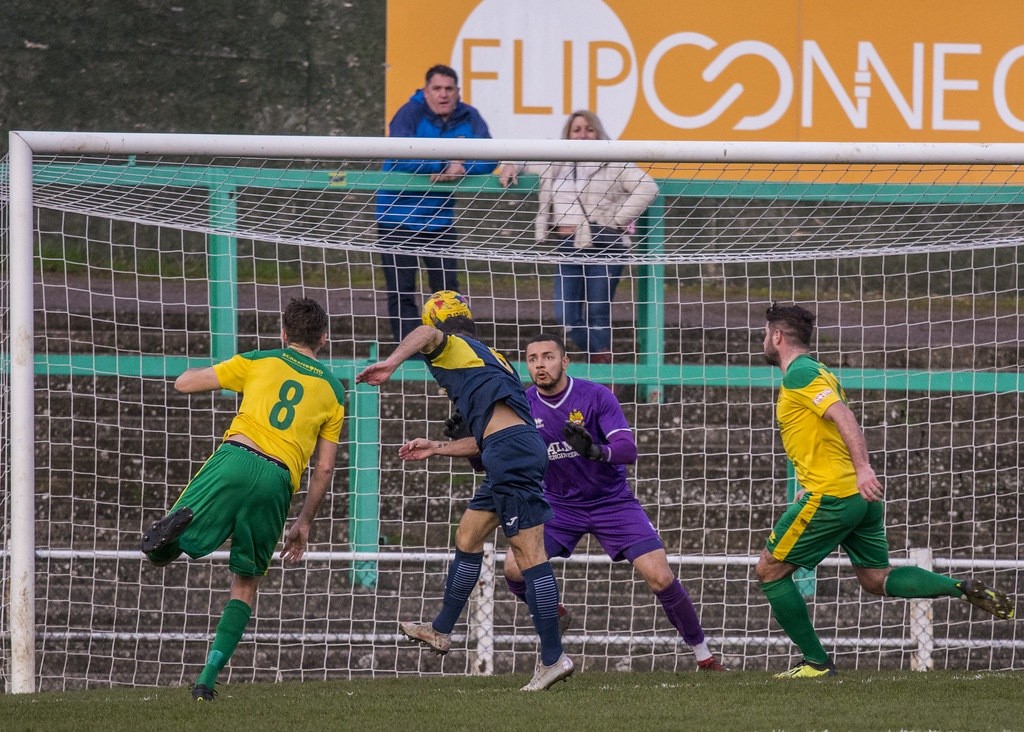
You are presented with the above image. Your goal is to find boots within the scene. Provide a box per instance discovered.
[586,348,628,399]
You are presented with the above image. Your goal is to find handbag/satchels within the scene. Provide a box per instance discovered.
[589,221,625,252]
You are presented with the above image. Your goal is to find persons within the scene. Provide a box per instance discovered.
[445,334,725,671]
[756,301,1020,678]
[140,297,347,701]
[496,111,658,397]
[354,289,574,692]
[375,64,501,362]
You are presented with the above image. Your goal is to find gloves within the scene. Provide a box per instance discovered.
[442,408,475,440]
[562,421,603,461]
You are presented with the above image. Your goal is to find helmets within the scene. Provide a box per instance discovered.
[421,290,474,334]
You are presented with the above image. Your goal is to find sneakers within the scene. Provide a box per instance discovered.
[954,578,1017,621]
[139,506,194,554]
[188,681,223,703]
[519,651,576,691]
[557,605,572,639]
[398,621,452,656]
[771,658,839,681]
[698,657,734,672]
